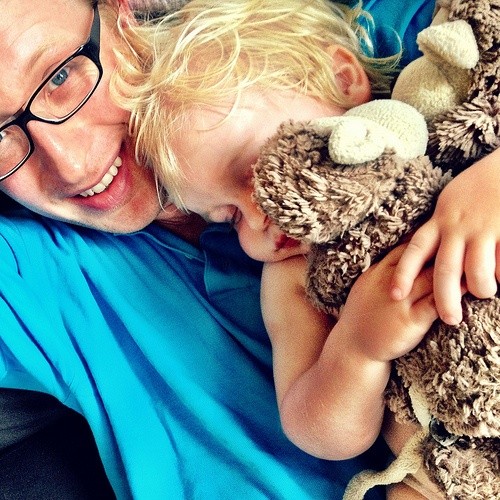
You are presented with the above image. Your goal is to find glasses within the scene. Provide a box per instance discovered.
[0,0,102,181]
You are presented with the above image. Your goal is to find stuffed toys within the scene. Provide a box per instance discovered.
[250,0,500,500]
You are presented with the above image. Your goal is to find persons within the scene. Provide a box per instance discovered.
[1,0,449,500]
[134,0,500,500]
